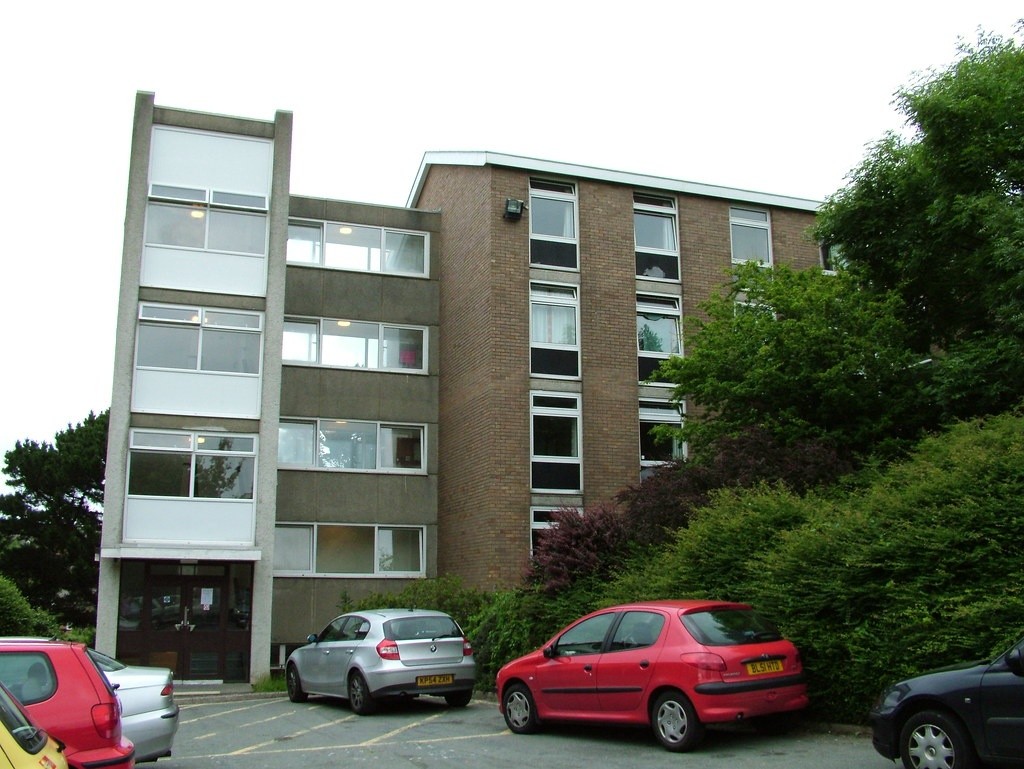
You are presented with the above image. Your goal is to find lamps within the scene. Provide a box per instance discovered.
[504,198,528,219]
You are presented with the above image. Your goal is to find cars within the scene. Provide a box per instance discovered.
[285,605,477,715]
[151,594,193,628]
[0,636,136,769]
[0,679,69,769]
[0,636,179,762]
[872,638,1024,769]
[497,601,808,751]
